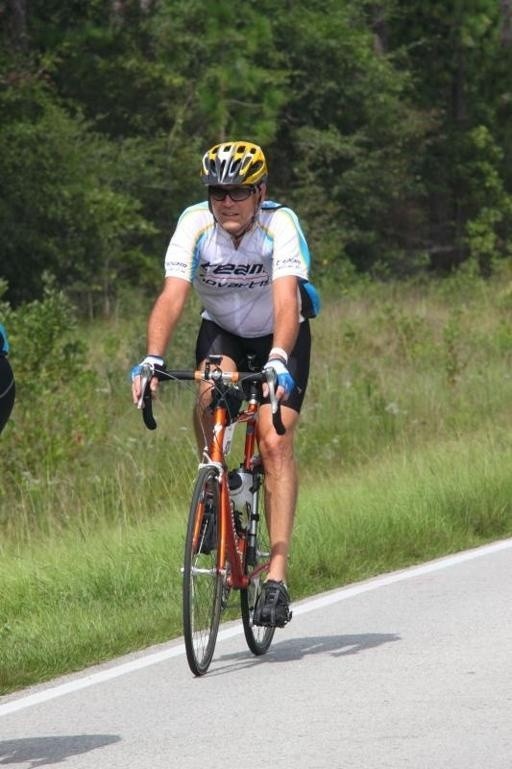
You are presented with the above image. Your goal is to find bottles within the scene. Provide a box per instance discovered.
[225,469,251,536]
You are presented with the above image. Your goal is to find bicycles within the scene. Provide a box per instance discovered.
[140,355,288,676]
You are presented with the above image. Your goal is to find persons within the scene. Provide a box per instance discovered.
[131,141,312,624]
[0,324,15,434]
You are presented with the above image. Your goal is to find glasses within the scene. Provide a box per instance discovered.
[211,188,255,200]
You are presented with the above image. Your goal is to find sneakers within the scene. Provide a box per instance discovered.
[254,580,292,627]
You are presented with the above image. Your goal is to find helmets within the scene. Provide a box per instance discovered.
[200,141,269,186]
